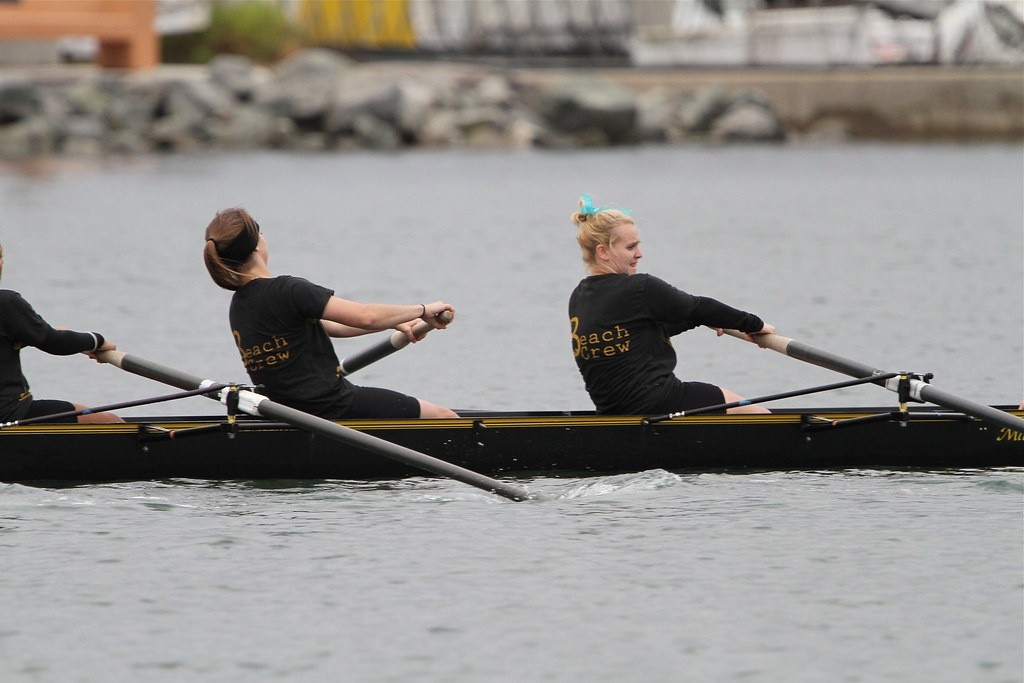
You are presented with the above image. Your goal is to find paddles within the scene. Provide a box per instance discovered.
[87,345,536,504]
[339,309,454,376]
[714,327,1023,436]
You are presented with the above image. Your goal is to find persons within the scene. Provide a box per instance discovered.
[568,197,778,416]
[0,247,125,423]
[203,208,466,419]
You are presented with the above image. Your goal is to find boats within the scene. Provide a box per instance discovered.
[1,407,1024,480]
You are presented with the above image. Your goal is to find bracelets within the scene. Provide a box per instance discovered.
[422,303,425,318]
